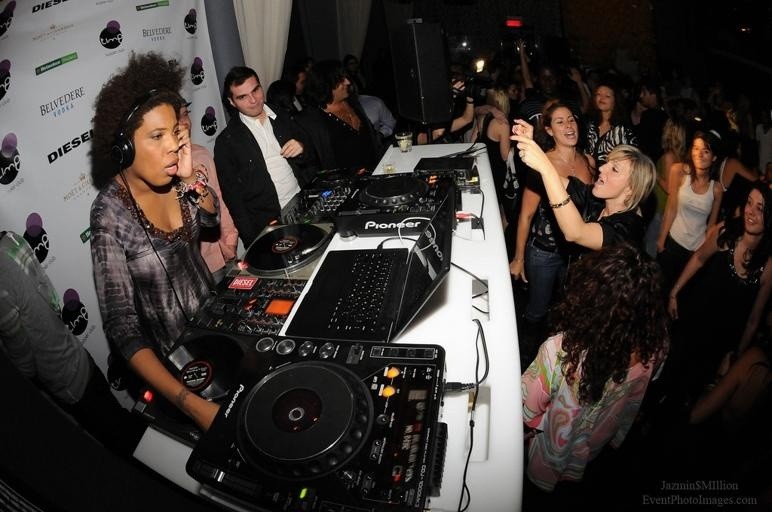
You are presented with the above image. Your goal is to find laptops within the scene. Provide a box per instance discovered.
[278,186,457,344]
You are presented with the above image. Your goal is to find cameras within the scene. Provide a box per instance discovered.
[514,128,522,136]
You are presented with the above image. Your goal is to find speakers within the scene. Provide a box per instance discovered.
[386,22,450,124]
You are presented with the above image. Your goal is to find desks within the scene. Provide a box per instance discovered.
[132,142,524,512]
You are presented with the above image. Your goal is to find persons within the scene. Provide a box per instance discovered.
[0,35,772,512]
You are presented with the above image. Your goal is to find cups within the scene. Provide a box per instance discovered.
[394,131,413,153]
[383,160,396,173]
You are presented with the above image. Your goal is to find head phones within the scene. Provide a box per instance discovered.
[104,87,170,172]
